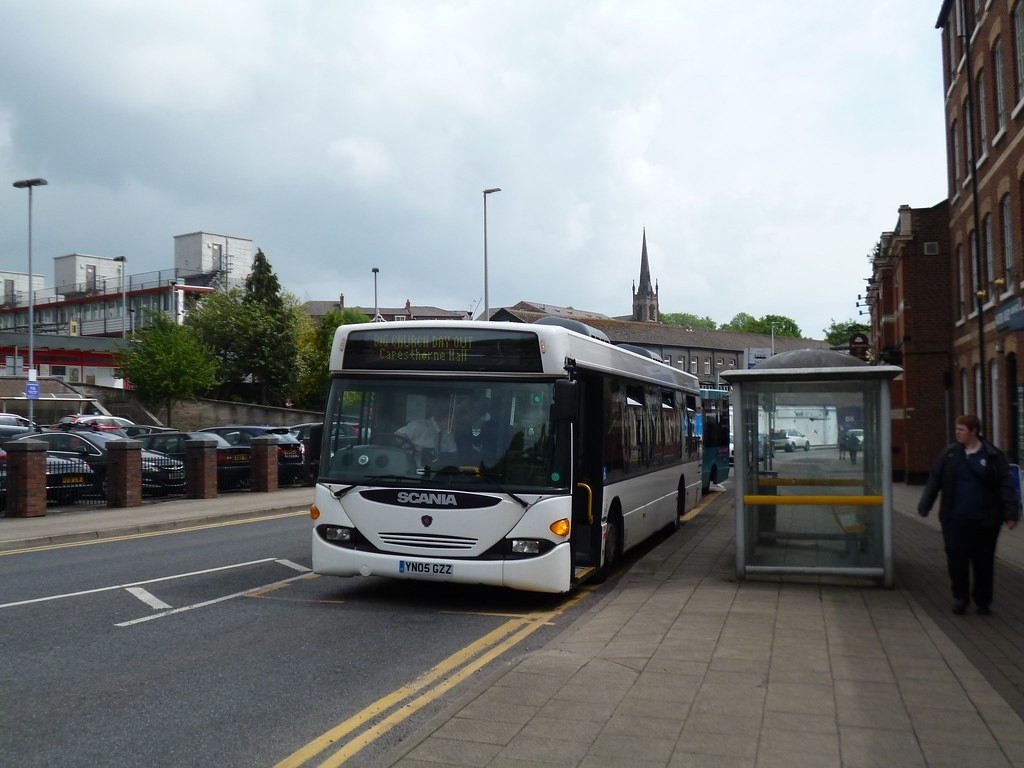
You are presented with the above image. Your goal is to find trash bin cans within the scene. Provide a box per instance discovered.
[757,471,778,533]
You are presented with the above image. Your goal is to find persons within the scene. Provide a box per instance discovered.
[838,429,859,464]
[455,401,521,464]
[396,404,457,465]
[919,414,1018,614]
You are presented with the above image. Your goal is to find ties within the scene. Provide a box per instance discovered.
[437,431,442,452]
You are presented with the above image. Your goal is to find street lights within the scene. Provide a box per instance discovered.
[371,267,380,322]
[13,177,47,436]
[770,321,781,359]
[482,187,502,319]
[114,255,128,392]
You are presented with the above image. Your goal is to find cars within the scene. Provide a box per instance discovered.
[845,429,865,451]
[772,429,812,452]
[727,431,775,464]
[0,412,363,505]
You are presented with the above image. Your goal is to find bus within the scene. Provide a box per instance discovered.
[700,389,733,493]
[312,314,705,598]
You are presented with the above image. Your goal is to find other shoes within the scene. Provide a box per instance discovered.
[976,603,992,616]
[953,593,971,614]
